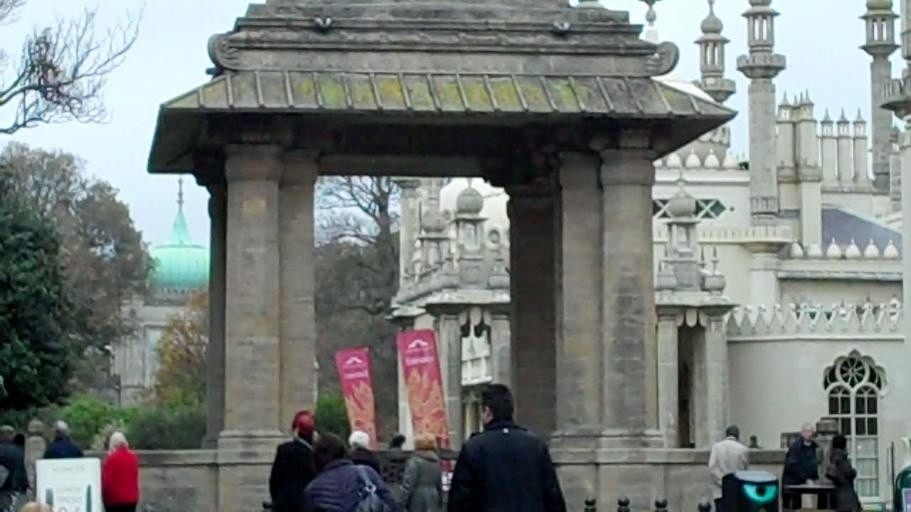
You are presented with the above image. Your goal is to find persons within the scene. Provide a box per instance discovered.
[267,410,321,512]
[0,419,140,512]
[446,384,568,512]
[302,432,404,512]
[347,430,382,476]
[749,435,763,449]
[707,425,750,512]
[400,432,443,512]
[780,424,823,512]
[824,434,863,511]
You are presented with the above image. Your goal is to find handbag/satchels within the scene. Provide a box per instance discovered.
[826,463,849,487]
[353,484,390,512]
